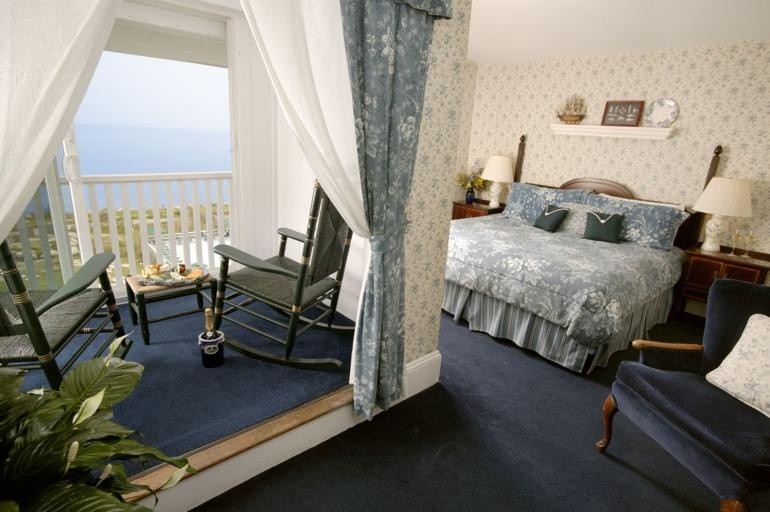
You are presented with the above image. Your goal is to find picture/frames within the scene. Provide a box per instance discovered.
[598,100,647,127]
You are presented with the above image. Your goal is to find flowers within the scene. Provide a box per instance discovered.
[0,327,199,511]
[456,165,495,197]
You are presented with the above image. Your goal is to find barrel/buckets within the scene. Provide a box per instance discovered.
[198,331,226,369]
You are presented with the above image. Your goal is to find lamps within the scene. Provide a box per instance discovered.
[478,152,515,212]
[690,176,754,254]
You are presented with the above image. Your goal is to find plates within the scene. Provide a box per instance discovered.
[644,98,680,129]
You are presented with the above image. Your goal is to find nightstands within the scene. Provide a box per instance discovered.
[666,246,770,322]
[450,198,507,220]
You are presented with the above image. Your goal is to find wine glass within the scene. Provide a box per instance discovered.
[728,226,743,258]
[743,229,758,259]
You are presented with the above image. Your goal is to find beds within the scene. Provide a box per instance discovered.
[441,133,724,381]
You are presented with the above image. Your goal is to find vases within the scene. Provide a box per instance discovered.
[466,187,476,205]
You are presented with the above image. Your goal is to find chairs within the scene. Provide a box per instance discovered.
[208,178,358,375]
[593,276,770,511]
[1,233,135,399]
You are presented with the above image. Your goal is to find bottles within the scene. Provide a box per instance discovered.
[202,306,218,339]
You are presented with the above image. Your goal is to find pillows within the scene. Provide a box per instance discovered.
[586,191,689,252]
[499,181,588,234]
[704,309,770,423]
[533,202,571,234]
[582,209,626,245]
[598,193,685,213]
